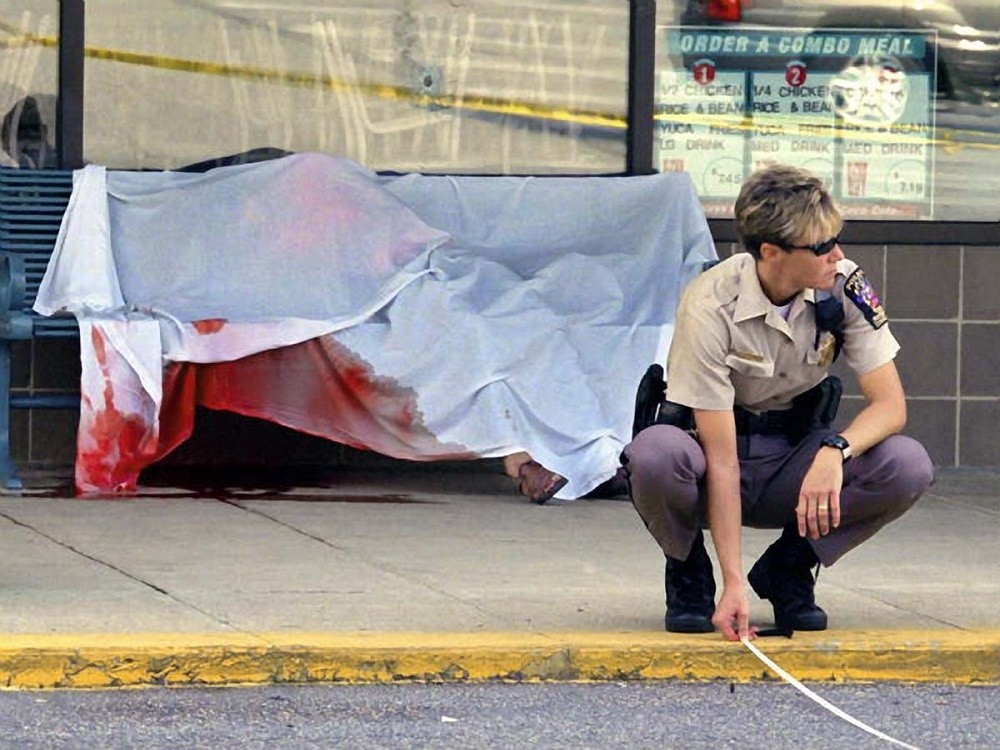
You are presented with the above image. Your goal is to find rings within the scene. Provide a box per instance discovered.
[817,505,828,511]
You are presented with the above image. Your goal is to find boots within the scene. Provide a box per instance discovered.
[664,532,715,632]
[747,525,827,630]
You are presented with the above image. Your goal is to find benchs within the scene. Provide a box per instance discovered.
[0,151,721,490]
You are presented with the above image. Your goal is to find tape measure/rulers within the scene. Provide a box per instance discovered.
[744,641,918,750]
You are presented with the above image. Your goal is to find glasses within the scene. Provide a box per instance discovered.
[790,233,841,256]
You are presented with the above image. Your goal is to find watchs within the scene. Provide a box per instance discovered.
[820,433,852,464]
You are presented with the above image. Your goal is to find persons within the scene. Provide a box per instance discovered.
[623,164,933,642]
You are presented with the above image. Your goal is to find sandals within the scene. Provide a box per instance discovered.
[512,460,569,504]
